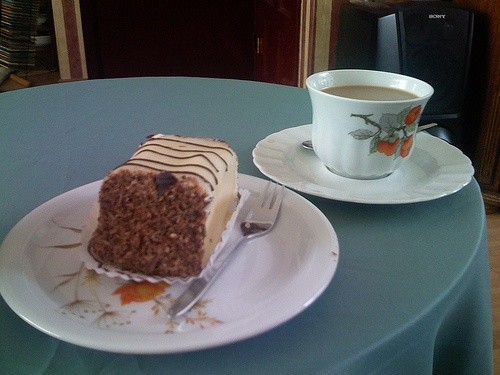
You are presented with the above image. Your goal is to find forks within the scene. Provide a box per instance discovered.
[166,179,286,319]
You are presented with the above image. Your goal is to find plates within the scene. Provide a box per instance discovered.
[253,122,474,203]
[0,173,339,355]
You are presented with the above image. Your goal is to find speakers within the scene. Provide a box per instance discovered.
[369,3,489,162]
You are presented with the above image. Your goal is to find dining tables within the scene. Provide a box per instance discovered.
[0,77,493,375]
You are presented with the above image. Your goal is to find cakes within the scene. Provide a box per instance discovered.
[87,133,239,277]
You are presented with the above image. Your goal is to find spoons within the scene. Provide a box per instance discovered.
[302,121,437,150]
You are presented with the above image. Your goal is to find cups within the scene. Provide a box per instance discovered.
[304,69,435,181]
